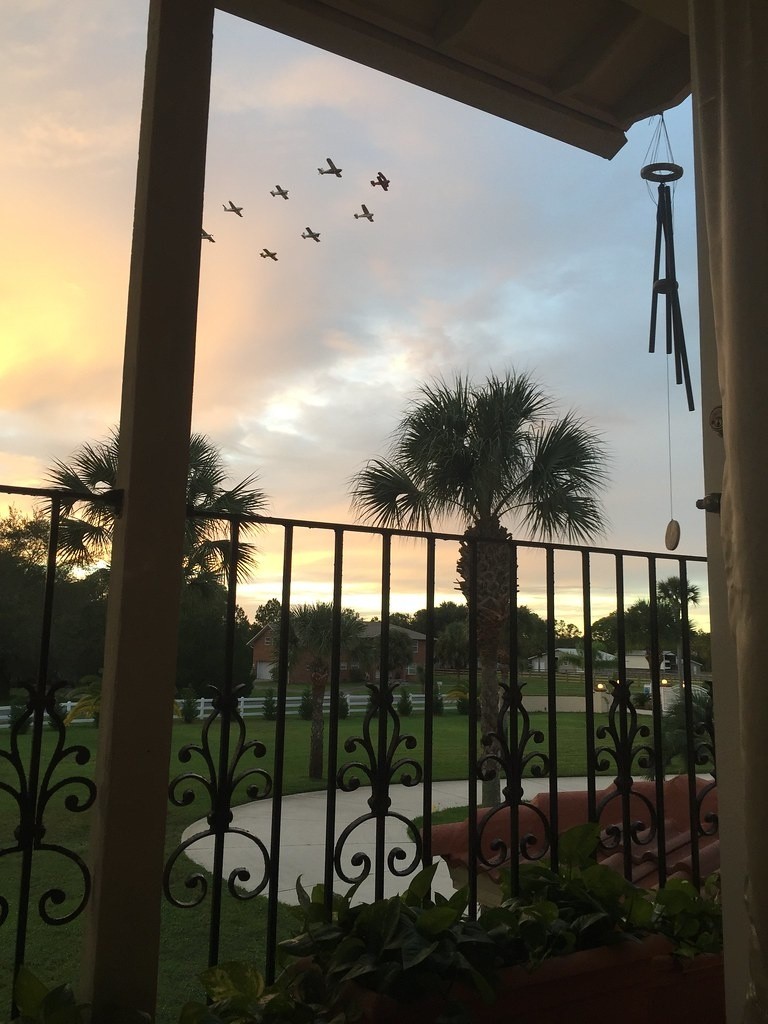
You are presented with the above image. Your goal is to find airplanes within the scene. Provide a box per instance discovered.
[353,204,374,222]
[260,248,278,261]
[221,200,243,217]
[202,229,216,243]
[377,172,390,183]
[301,227,320,242]
[269,185,289,200]
[371,176,388,191]
[317,158,342,178]
